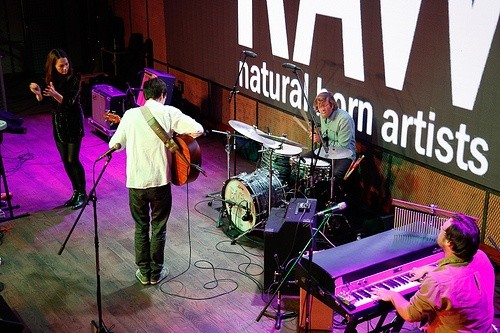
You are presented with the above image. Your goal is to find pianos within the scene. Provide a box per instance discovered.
[292,221,445,333]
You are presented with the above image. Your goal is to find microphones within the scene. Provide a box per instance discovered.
[242,50,258,58]
[242,214,252,221]
[281,62,303,70]
[96,143,121,161]
[312,202,347,217]
[130,88,144,91]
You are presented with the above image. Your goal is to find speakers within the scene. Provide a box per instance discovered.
[262,198,318,293]
[136,67,177,107]
[92,84,126,130]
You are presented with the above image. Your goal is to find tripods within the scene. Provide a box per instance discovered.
[256,212,330,330]
[229,145,280,243]
[207,130,247,225]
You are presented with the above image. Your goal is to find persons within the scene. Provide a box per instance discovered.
[107,78,206,285]
[369,212,495,333]
[302,92,353,196]
[29,47,89,210]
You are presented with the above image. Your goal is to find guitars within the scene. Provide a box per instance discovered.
[103,109,202,185]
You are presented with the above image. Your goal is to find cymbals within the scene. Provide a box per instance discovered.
[288,157,331,168]
[315,145,353,159]
[228,120,275,144]
[258,132,310,150]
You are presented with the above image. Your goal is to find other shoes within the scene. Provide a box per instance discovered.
[136,269,150,285]
[150,267,169,284]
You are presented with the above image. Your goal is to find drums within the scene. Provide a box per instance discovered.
[222,167,285,233]
[289,157,331,181]
[259,143,303,179]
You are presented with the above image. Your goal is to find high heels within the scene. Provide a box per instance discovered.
[64,191,79,206]
[72,193,88,209]
